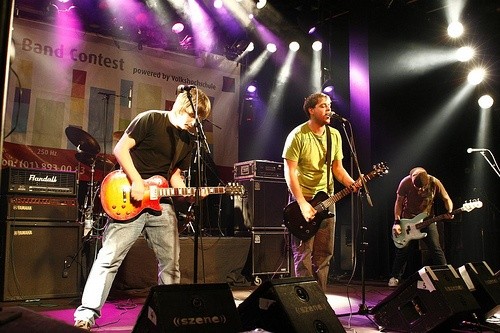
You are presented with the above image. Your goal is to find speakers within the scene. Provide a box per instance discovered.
[242,230,291,275]
[130,281,240,333]
[370,263,482,333]
[0,306,99,333]
[237,275,350,333]
[230,179,290,230]
[455,262,500,333]
[0,220,85,301]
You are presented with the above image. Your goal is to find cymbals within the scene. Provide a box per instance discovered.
[75,153,116,171]
[65,127,101,154]
[113,131,124,140]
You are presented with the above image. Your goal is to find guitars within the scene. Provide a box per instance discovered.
[391,198,483,249]
[283,162,390,241]
[101,170,246,221]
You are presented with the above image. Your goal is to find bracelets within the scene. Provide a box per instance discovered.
[394,220,400,224]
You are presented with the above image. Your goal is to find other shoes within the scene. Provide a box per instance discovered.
[388,277,399,287]
[74,319,90,332]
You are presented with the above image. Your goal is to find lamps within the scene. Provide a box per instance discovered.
[247,79,257,92]
[322,64,334,93]
[309,27,325,42]
[171,17,184,33]
[43,0,78,12]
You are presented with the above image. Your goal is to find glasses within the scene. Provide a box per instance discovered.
[183,109,195,119]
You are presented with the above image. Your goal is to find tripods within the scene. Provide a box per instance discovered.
[333,119,382,328]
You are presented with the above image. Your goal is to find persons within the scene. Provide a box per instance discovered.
[389,167,454,286]
[74,87,212,330]
[282,92,361,293]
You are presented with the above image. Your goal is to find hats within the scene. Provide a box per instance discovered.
[409,167,428,186]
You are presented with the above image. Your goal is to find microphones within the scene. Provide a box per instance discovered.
[329,112,347,122]
[466,147,488,153]
[177,84,195,93]
[62,258,69,277]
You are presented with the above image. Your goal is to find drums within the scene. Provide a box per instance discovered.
[141,195,192,234]
[85,183,108,218]
